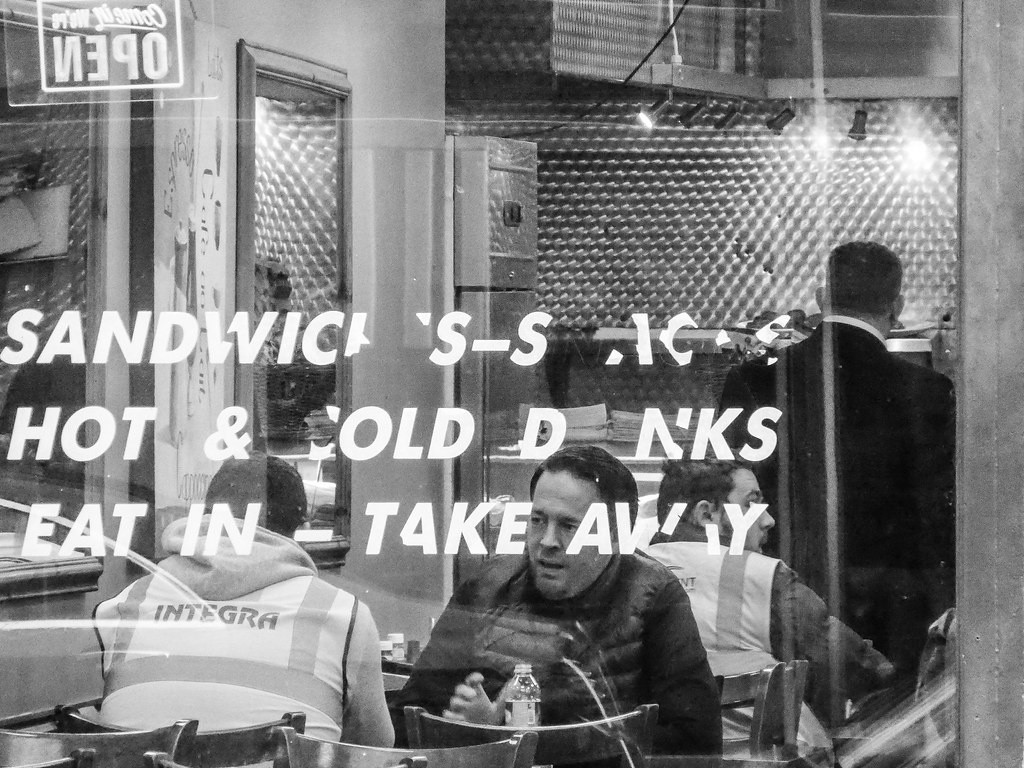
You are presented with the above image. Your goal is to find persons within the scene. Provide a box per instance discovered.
[389,447,724,767]
[716,241,955,681]
[636,450,901,767]
[100,455,395,749]
[255,250,336,442]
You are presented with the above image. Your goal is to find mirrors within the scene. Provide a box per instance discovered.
[229,38,356,569]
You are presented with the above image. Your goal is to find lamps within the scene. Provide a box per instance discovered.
[629,81,869,145]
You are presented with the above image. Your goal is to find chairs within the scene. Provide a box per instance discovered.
[0,647,816,767]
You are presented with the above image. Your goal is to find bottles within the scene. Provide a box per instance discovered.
[388,633,406,663]
[504,663,540,728]
[380,641,393,662]
